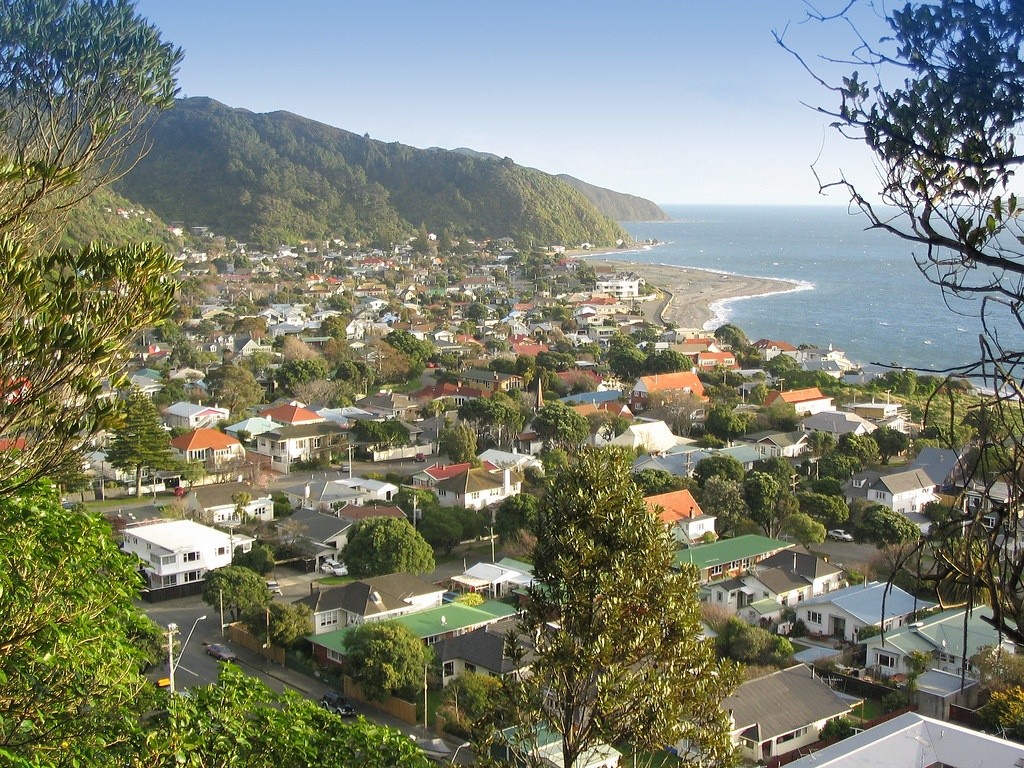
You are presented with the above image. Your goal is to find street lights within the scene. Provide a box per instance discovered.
[167,615,207,699]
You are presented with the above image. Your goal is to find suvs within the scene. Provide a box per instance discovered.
[319,693,356,719]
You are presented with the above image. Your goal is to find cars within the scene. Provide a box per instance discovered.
[416,453,425,462]
[322,560,348,577]
[341,463,349,472]
[206,643,236,662]
[175,487,185,496]
[827,529,853,542]
[265,581,279,593]
